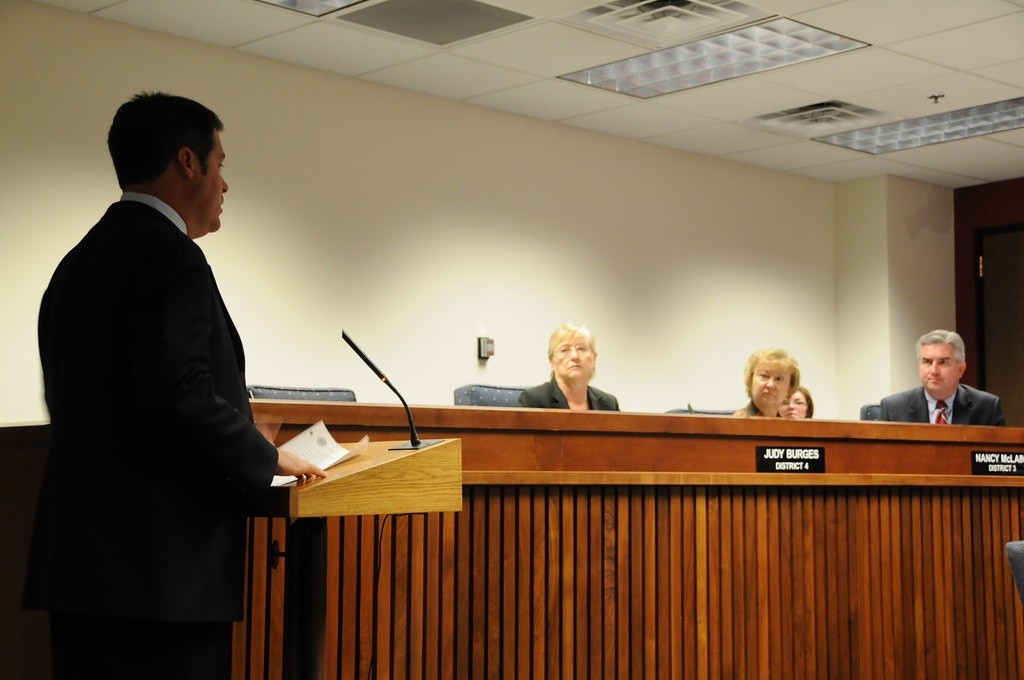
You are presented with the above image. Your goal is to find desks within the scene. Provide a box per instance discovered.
[232,403,1024,680]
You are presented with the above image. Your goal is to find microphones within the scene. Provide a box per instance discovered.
[342,329,421,446]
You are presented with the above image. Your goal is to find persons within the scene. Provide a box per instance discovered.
[730,347,799,418]
[777,385,814,418]
[38,92,327,680]
[520,324,621,411]
[881,330,1003,424]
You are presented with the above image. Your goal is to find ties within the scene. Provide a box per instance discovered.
[936,400,949,424]
